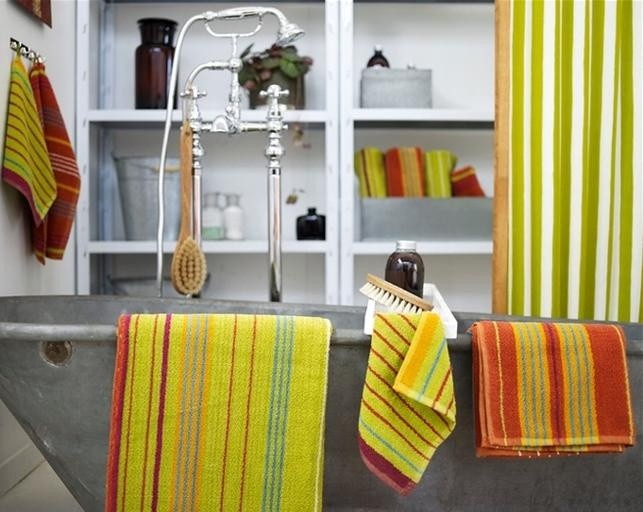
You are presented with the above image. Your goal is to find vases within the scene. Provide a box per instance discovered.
[248,66,305,110]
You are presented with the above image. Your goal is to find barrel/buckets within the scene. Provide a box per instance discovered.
[111,152,189,241]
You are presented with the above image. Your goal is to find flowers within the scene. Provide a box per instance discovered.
[236,41,314,91]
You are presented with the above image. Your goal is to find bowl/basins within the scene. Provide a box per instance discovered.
[361,66,433,111]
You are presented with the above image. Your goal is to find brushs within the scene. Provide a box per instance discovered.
[171,120,207,295]
[358,273,434,315]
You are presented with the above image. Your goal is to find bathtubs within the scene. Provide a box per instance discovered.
[0,295,642,512]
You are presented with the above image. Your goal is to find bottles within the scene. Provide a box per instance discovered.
[367,46,390,67]
[133,16,176,110]
[199,190,242,241]
[295,208,324,240]
[385,240,425,299]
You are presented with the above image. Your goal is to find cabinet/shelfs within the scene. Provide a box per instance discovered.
[340,0,507,316]
[73,0,339,306]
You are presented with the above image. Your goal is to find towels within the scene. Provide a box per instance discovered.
[1,42,59,267]
[357,310,459,498]
[26,61,82,261]
[464,318,636,462]
[103,311,333,512]
